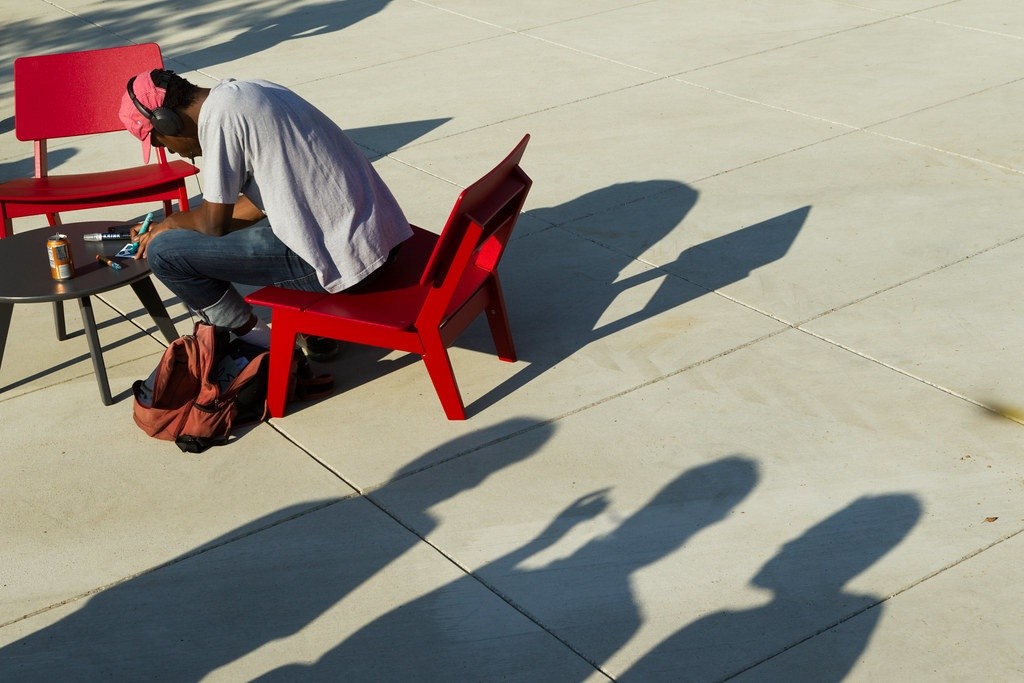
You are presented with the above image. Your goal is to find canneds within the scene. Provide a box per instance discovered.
[47,232,75,280]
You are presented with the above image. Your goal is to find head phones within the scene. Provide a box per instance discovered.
[127,75,184,137]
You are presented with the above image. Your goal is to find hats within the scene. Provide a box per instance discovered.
[118,67,174,166]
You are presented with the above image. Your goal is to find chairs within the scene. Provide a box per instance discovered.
[242,133,533,422]
[0,42,201,238]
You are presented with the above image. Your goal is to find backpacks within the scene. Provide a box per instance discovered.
[131,322,272,455]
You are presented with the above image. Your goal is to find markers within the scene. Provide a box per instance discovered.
[131,212,153,252]
[96,255,122,270]
[84,232,131,241]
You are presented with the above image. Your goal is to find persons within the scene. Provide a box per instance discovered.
[118,69,414,383]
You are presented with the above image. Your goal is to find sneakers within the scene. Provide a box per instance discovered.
[303,335,339,363]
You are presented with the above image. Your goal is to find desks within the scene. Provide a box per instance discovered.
[1,221,181,407]
